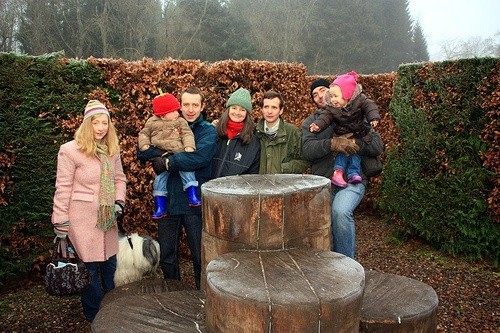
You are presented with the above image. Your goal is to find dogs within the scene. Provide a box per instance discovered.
[113,231,160,288]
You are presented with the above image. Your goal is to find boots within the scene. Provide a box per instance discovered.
[183,181,202,206]
[348,173,362,183]
[151,190,168,219]
[331,166,347,188]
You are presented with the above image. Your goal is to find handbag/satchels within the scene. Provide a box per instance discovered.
[360,156,383,178]
[44,236,93,296]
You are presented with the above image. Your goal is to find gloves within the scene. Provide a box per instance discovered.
[149,147,169,175]
[53,224,69,239]
[114,201,125,216]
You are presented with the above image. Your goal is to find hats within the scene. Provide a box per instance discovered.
[311,78,331,106]
[152,93,181,115]
[226,87,252,113]
[333,71,360,100]
[84,99,110,119]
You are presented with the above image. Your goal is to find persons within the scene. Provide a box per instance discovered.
[310,71,381,187]
[51,100,127,322]
[302,80,383,259]
[137,86,217,290]
[138,93,202,219]
[211,88,261,179]
[254,91,310,174]
